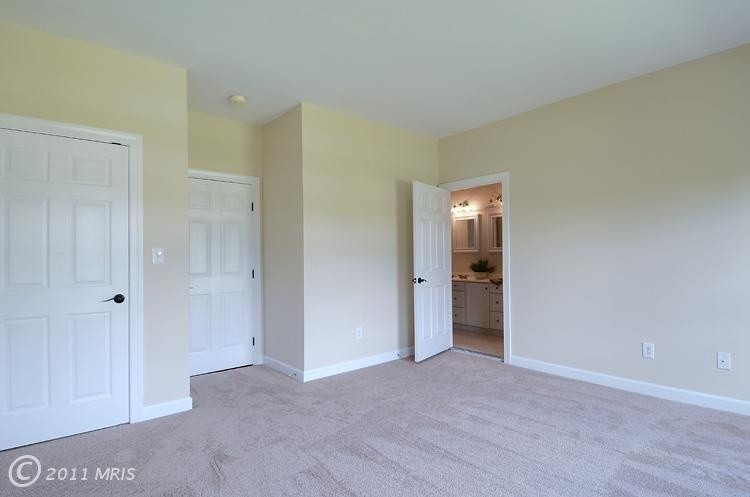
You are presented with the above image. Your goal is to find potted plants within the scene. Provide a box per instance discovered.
[469,255,498,278]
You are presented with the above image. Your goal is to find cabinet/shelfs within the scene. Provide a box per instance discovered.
[452,281,503,337]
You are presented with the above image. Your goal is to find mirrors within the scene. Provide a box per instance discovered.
[453,214,480,253]
[486,211,502,253]
